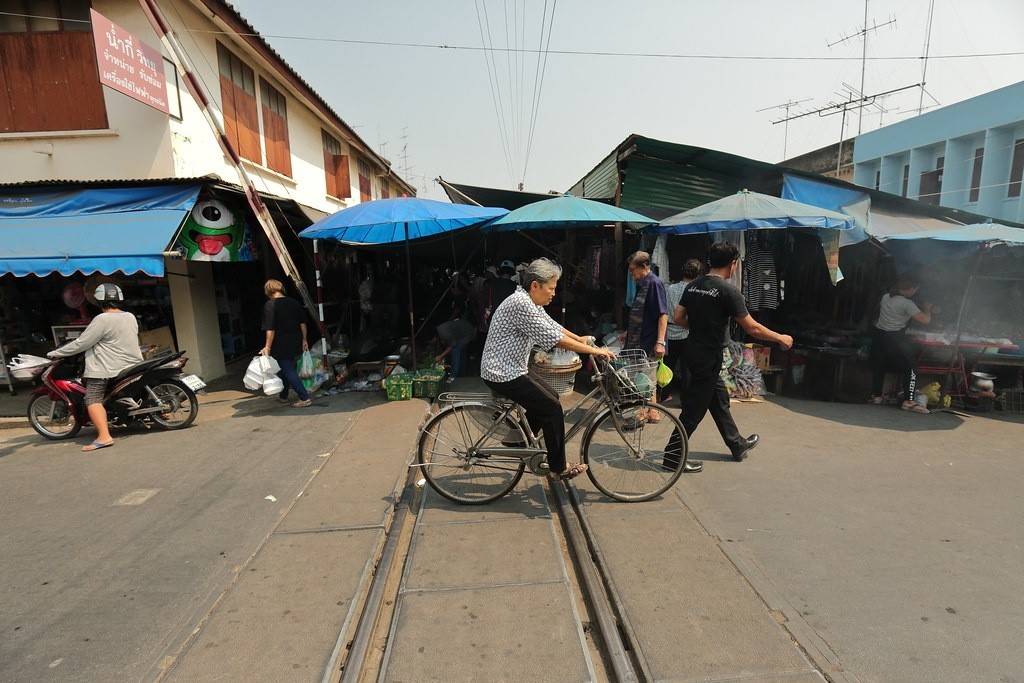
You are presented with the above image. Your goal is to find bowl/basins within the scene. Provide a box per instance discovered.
[244,355,283,395]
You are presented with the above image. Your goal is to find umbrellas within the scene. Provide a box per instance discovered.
[868,218,1024,408]
[481,190,661,327]
[299,193,509,368]
[642,189,857,237]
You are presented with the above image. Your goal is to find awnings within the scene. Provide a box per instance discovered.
[0,186,202,278]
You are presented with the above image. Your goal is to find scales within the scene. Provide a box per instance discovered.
[398,337,412,356]
[385,355,406,376]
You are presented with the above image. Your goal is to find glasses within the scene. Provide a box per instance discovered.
[630,264,639,272]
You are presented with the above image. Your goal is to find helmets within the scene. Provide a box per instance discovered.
[94,283,125,302]
[501,260,515,270]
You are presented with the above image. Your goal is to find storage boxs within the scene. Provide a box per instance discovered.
[385,370,449,400]
[243,354,284,397]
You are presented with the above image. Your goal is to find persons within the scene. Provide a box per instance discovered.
[874,275,932,413]
[618,240,793,472]
[481,257,617,484]
[259,279,313,407]
[424,256,519,383]
[47,283,144,452]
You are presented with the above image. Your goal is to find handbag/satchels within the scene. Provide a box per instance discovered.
[478,304,495,332]
[298,348,315,379]
[7,353,51,380]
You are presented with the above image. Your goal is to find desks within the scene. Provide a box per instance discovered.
[905,336,1020,409]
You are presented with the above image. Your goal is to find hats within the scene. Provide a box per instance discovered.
[486,266,500,278]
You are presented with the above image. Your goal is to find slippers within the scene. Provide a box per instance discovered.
[83,440,115,451]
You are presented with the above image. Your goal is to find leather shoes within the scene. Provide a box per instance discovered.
[731,434,759,462]
[663,459,705,473]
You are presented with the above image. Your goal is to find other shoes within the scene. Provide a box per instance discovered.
[901,401,930,414]
[620,412,660,431]
[871,394,882,404]
[552,463,590,481]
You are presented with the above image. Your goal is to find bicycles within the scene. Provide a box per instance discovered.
[407,336,690,506]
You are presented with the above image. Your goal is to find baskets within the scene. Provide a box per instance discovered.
[10,360,54,382]
[603,348,660,404]
[532,366,576,396]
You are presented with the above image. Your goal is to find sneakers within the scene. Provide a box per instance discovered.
[278,396,289,403]
[290,399,312,407]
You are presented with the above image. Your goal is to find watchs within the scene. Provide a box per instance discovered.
[656,341,666,346]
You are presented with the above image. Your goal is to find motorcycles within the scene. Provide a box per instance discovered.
[26,337,198,440]
